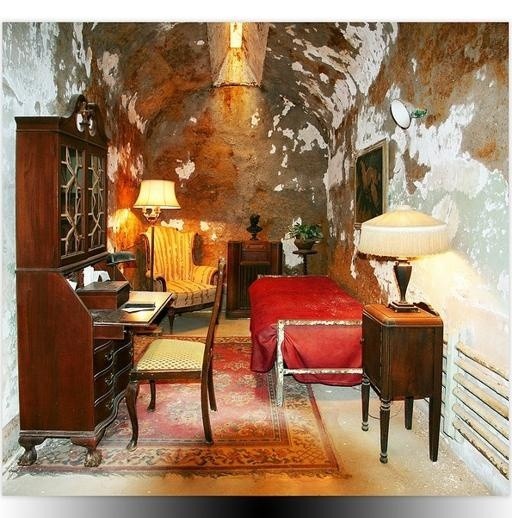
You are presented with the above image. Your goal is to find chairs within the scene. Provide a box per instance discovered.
[137,224,223,328]
[123,257,233,452]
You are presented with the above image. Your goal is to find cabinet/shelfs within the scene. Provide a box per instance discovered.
[13,252,172,441]
[14,93,110,271]
[359,299,445,465]
[227,238,283,319]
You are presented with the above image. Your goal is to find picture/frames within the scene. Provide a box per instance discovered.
[352,136,393,230]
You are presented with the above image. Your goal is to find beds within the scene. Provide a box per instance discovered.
[247,272,377,408]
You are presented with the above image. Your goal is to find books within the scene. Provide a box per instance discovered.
[124,301,155,310]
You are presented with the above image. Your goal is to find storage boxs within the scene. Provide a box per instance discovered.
[76,281,130,311]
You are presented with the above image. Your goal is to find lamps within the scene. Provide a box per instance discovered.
[133,177,182,290]
[390,95,429,128]
[356,207,456,312]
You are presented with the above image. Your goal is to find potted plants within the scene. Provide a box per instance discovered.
[287,220,325,251]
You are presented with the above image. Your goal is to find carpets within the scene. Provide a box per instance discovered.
[10,329,344,479]
